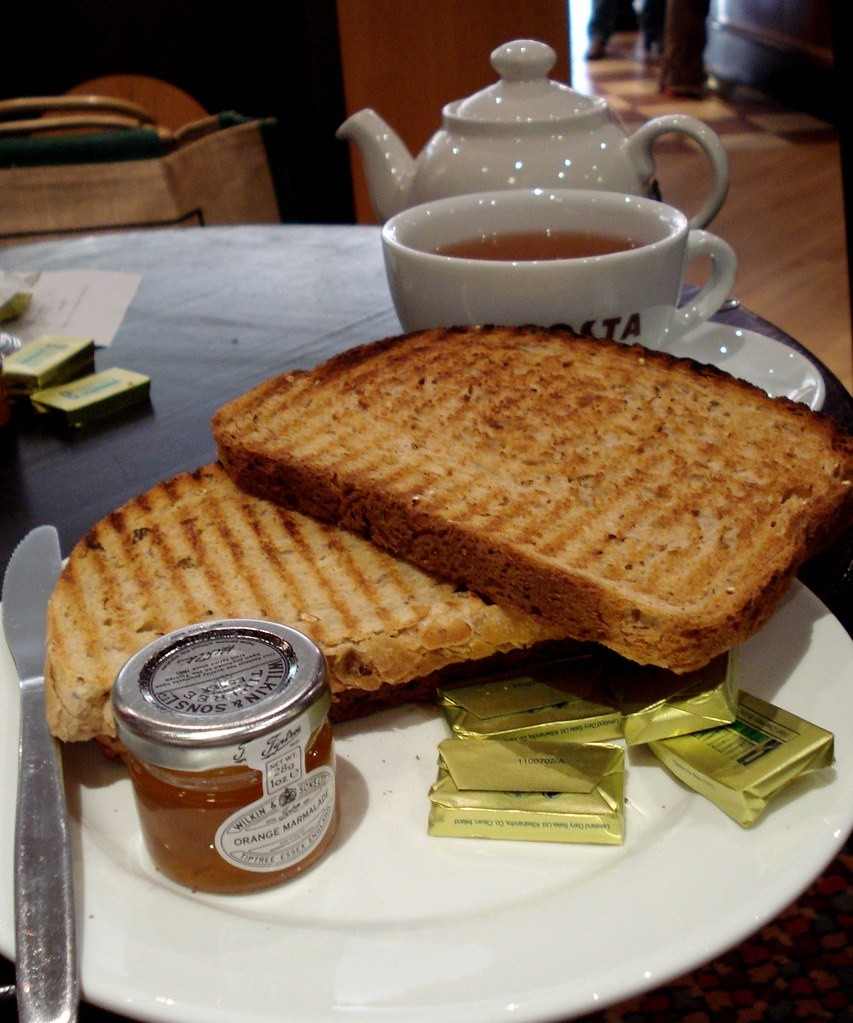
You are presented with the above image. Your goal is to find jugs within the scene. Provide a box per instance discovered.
[337,38,730,228]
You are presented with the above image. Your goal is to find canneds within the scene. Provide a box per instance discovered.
[109,619,341,894]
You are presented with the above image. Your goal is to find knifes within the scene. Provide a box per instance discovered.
[1,525,80,1023]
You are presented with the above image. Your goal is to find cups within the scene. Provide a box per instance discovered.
[382,187,737,354]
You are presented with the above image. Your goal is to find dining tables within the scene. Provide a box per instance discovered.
[0,222,853,1023]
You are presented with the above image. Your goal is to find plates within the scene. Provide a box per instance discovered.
[666,322,828,415]
[1,557,853,1023]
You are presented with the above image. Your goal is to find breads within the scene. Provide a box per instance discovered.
[210,321,853,675]
[42,457,562,743]
